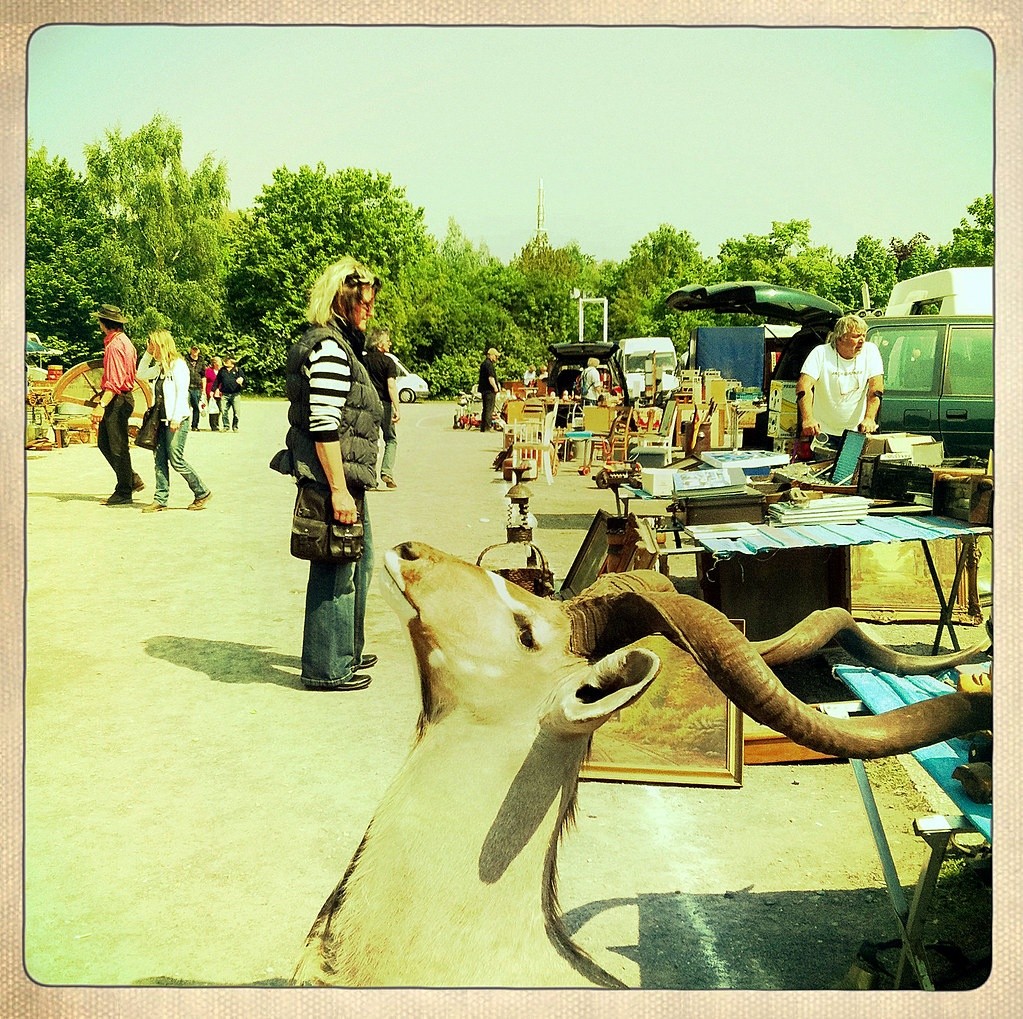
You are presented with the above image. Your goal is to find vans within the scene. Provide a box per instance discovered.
[544,341,631,428]
[663,266,994,460]
[362,351,430,404]
[616,337,679,400]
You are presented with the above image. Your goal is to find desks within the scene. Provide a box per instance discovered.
[618,483,674,518]
[824,665,992,991]
[659,515,993,655]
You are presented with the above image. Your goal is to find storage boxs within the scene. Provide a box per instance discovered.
[737,409,756,428]
[767,379,798,454]
[862,432,936,477]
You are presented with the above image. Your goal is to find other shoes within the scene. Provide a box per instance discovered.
[142,501,167,513]
[106,491,132,505]
[366,483,377,491]
[132,473,145,495]
[188,491,213,510]
[381,474,397,488]
[487,426,495,433]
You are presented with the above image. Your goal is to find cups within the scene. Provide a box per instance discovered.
[810,432,829,451]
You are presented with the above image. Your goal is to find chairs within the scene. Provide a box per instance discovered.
[512,400,678,486]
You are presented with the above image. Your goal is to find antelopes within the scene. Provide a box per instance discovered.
[295,535,993,985]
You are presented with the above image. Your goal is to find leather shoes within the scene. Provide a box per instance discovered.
[359,654,379,669]
[305,674,372,690]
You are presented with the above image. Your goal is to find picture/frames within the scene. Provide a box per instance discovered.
[560,508,613,600]
[742,712,842,765]
[578,619,745,787]
[977,535,993,606]
[850,536,983,626]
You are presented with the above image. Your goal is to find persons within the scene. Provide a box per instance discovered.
[478,348,500,432]
[136,331,213,514]
[523,365,537,387]
[795,315,885,460]
[539,364,548,379]
[270,255,384,691]
[88,304,146,506]
[581,357,602,405]
[204,356,223,431]
[184,345,206,431]
[361,329,401,491]
[209,356,245,433]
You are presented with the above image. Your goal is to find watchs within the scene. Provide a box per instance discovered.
[99,401,107,408]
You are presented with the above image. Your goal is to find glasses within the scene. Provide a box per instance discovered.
[837,377,859,396]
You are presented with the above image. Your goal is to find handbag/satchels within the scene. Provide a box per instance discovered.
[235,366,249,391]
[291,476,365,560]
[134,405,161,451]
[208,397,220,414]
[198,394,207,417]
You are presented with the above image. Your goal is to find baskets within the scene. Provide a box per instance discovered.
[477,541,555,597]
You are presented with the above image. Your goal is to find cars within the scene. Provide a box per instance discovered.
[25,356,48,382]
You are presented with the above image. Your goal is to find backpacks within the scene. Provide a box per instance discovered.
[579,368,594,396]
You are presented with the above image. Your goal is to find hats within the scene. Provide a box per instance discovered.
[191,344,200,351]
[89,303,129,325]
[488,348,502,356]
